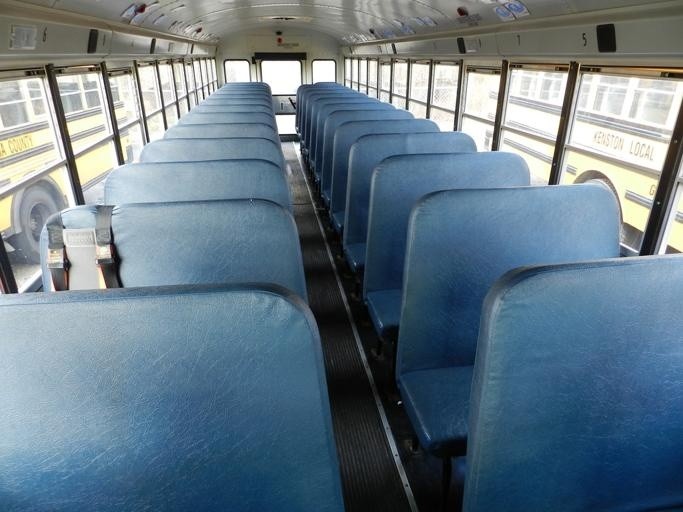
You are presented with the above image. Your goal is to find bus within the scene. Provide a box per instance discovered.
[484,71,682,254]
[0,75,132,262]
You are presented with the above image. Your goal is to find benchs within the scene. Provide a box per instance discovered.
[2,82,682,510]
